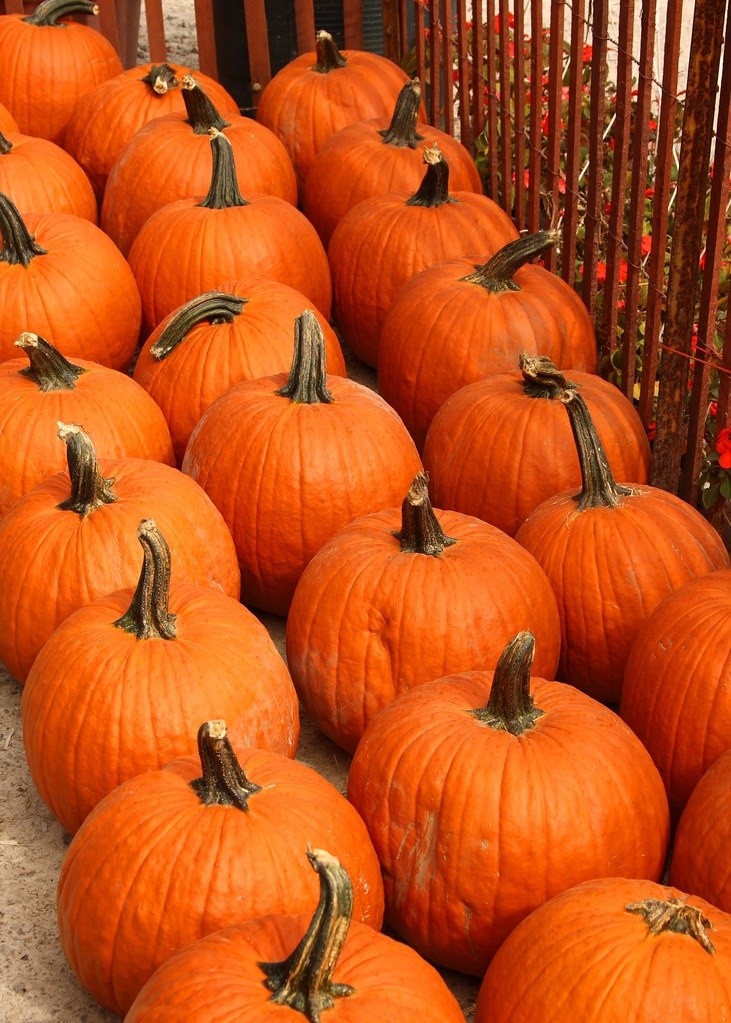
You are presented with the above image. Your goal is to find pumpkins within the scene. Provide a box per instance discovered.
[0,0,731,1023]
